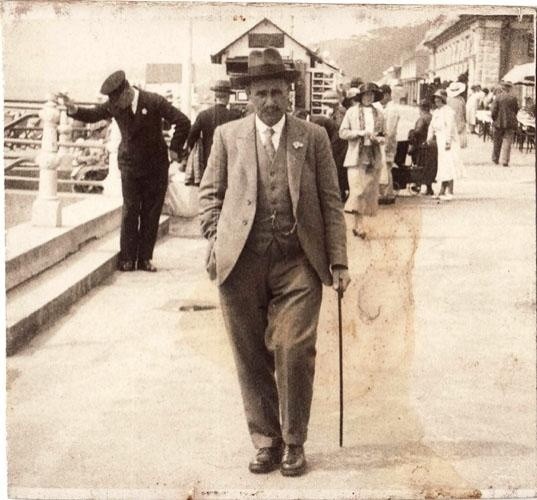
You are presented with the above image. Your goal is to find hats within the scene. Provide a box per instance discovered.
[446,82,465,97]
[230,48,301,89]
[353,82,384,103]
[210,80,236,94]
[502,80,513,86]
[99,70,127,96]
[430,89,447,104]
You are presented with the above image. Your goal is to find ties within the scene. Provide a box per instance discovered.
[262,129,275,160]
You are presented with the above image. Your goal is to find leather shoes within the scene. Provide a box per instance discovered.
[353,229,366,238]
[119,261,134,271]
[281,445,305,475]
[379,199,395,204]
[425,190,433,195]
[412,186,420,193]
[249,447,281,473]
[137,261,156,271]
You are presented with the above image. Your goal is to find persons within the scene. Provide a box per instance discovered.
[196,46,352,478]
